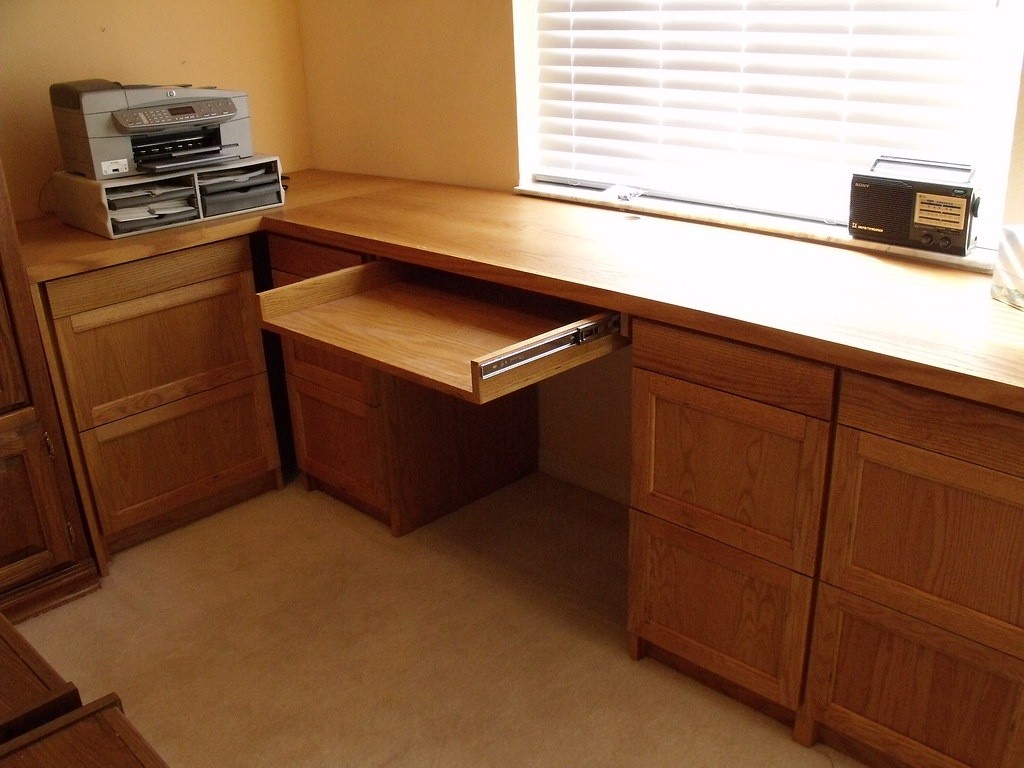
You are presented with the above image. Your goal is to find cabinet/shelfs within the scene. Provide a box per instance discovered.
[264,234,537,535]
[625,318,1024,768]
[0,157,101,626]
[44,237,284,556]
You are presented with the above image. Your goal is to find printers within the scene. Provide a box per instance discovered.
[52,78,253,182]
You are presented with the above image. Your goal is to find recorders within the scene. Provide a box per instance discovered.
[848,155,981,256]
